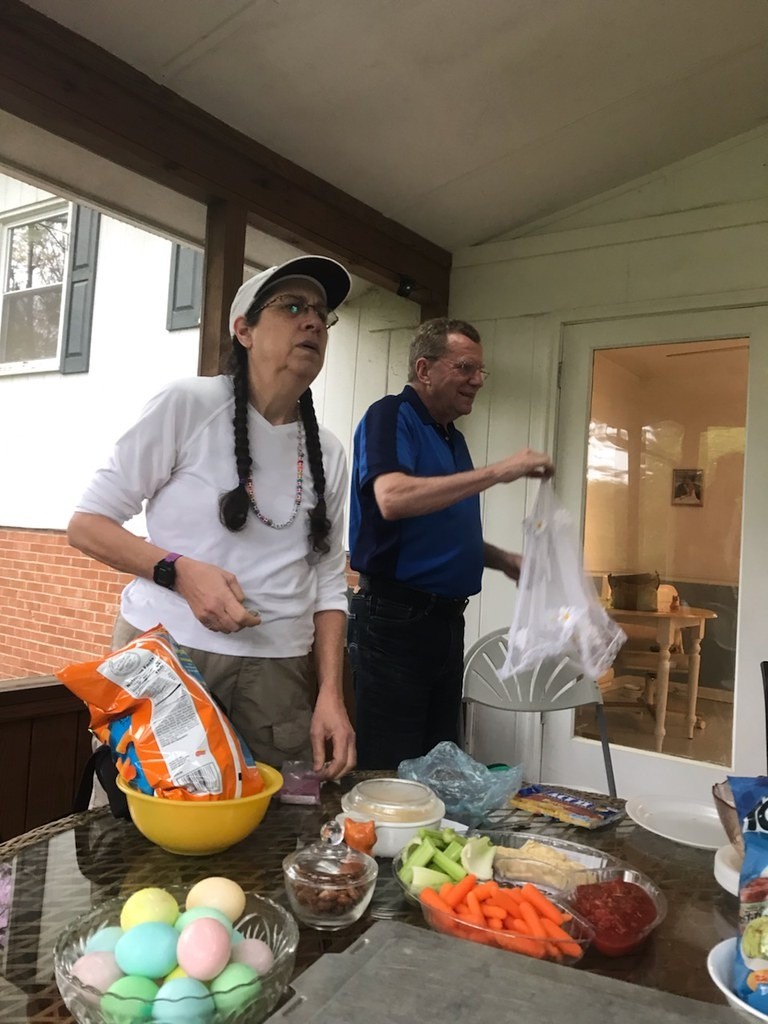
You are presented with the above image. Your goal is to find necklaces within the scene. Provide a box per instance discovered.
[247,398,304,529]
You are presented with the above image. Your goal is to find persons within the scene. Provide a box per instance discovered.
[66,251,359,818]
[346,315,554,770]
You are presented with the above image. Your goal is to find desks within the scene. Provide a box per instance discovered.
[0,767,768,1024]
[604,607,718,754]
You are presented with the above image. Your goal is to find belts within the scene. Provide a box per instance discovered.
[358,572,469,618]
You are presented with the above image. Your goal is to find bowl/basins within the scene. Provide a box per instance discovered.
[117,761,283,855]
[53,884,298,1024]
[341,778,445,858]
[708,937,768,1024]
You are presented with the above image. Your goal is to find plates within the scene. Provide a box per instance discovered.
[625,794,733,850]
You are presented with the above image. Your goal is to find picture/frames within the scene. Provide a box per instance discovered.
[670,468,705,508]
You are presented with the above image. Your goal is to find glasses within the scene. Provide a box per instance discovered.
[434,359,490,381]
[254,294,338,330]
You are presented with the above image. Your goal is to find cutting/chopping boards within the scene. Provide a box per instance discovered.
[265,920,747,1024]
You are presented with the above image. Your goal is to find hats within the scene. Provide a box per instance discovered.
[228,255,352,341]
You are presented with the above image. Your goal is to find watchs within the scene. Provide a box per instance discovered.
[152,552,184,592]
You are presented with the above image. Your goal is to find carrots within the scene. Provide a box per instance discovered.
[418,873,583,967]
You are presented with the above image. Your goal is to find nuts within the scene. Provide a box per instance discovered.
[293,869,363,916]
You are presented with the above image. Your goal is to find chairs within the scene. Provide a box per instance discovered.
[600,576,706,729]
[461,627,619,797]
